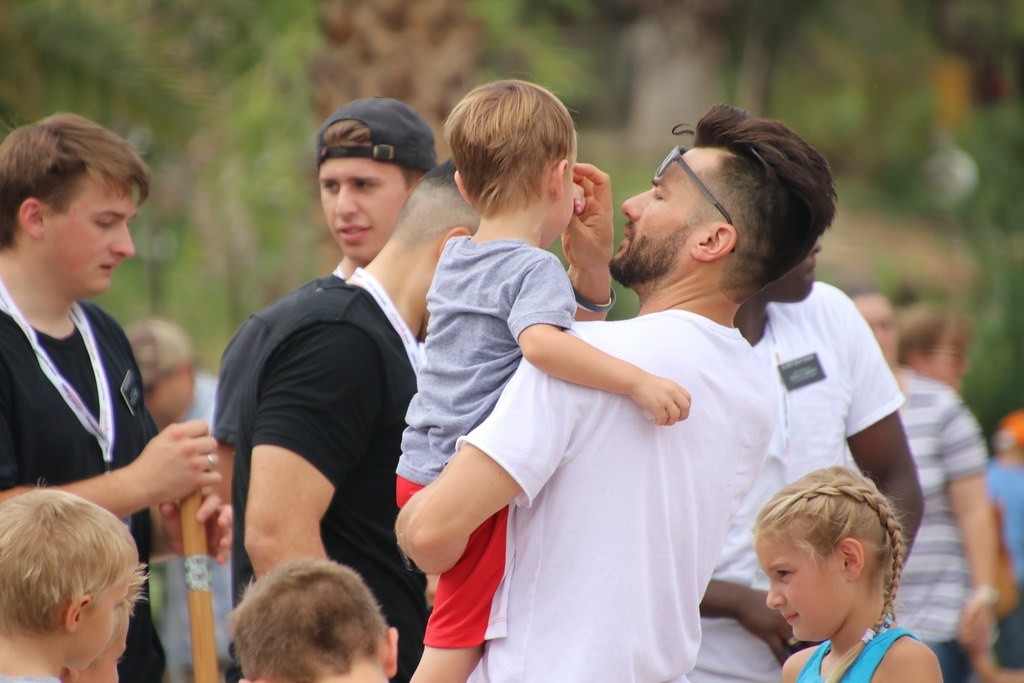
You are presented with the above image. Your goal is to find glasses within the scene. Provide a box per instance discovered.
[655,145,735,252]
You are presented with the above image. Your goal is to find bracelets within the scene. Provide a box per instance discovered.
[569,278,620,314]
[974,582,999,605]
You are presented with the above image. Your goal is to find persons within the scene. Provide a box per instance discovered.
[120,311,237,683]
[228,153,484,683]
[689,232,926,683]
[395,99,844,683]
[208,94,437,512]
[0,110,234,683]
[60,564,150,683]
[0,478,140,683]
[754,466,943,683]
[848,288,1024,683]
[387,78,692,683]
[228,558,399,683]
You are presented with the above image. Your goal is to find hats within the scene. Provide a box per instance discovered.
[998,411,1024,447]
[316,96,438,169]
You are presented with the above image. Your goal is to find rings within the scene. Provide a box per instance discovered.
[207,454,214,466]
